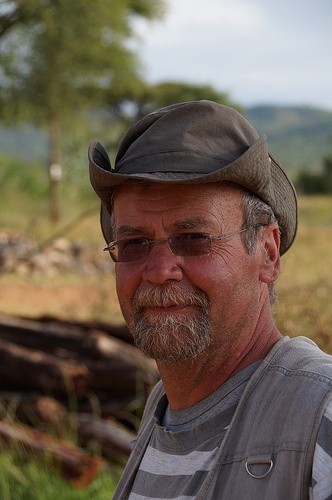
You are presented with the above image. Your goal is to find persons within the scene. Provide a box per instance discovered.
[89,100,332,500]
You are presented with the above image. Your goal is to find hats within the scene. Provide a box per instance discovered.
[88,99,298,257]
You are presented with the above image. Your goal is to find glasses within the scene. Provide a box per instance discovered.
[102,223,272,263]
